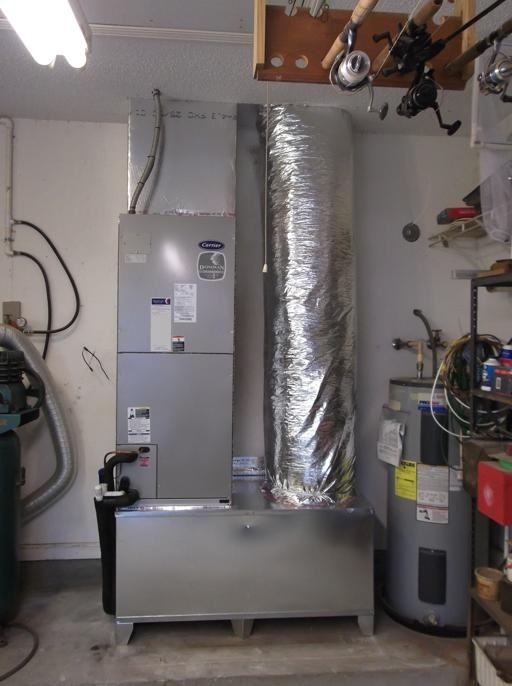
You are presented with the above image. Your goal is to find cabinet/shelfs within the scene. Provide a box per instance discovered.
[466,272,512,686]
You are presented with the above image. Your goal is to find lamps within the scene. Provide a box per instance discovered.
[0,0,95,70]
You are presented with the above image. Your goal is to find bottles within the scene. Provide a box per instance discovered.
[481,343,511,397]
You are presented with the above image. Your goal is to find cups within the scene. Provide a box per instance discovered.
[474,566,503,601]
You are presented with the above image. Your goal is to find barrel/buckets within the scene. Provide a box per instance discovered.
[383,378,499,638]
